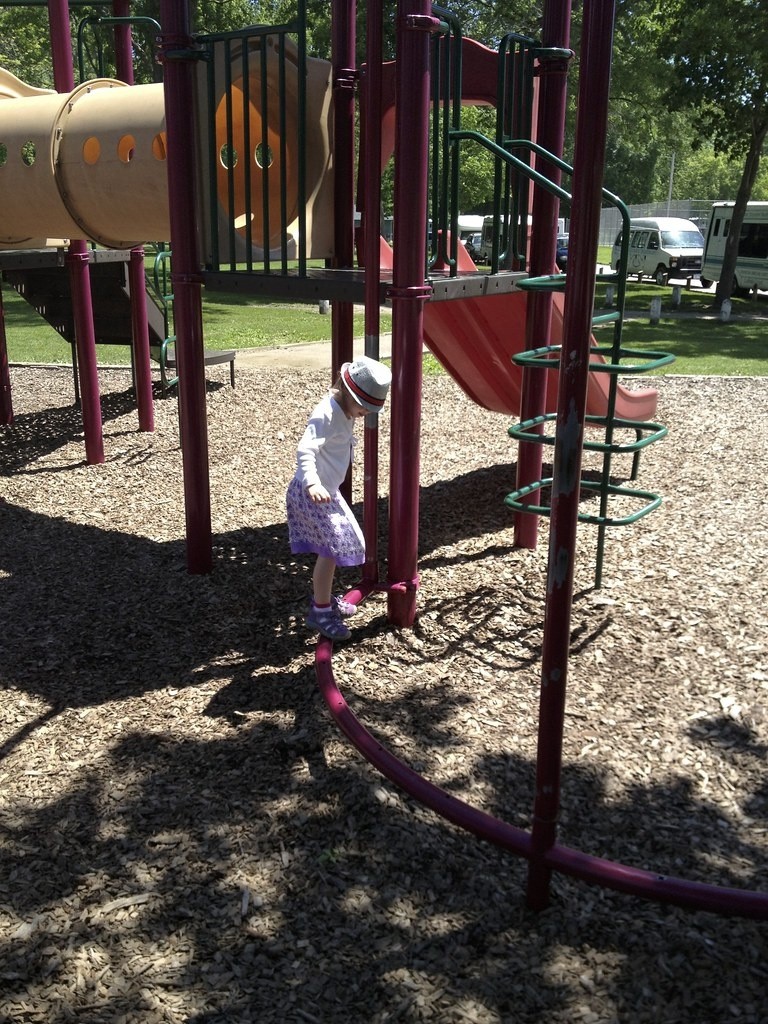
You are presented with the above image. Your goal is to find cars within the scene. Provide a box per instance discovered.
[463,233,486,263]
[556,237,569,273]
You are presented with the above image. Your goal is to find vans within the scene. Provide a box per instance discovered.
[610,217,706,287]
[480,214,533,266]
[701,201,767,298]
[457,215,485,247]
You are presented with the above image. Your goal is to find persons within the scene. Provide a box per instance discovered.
[285,355,393,640]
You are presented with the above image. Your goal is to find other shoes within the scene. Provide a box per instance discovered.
[311,594,357,617]
[306,608,352,640]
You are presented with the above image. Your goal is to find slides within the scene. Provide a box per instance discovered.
[368,228,658,426]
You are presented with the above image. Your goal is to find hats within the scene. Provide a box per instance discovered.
[340,356,392,413]
